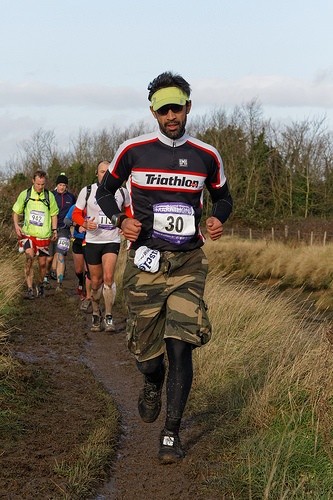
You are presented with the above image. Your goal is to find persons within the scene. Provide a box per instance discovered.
[63,204,91,311]
[43,175,77,291]
[72,160,134,332]
[12,170,59,299]
[94,71,233,461]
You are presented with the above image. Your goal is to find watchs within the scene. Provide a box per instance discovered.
[116,215,128,229]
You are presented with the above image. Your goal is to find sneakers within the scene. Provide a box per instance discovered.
[158,427,181,464]
[90,314,101,332]
[80,298,91,311]
[103,314,115,331]
[138,363,165,423]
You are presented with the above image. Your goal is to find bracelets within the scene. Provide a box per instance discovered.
[52,229,57,231]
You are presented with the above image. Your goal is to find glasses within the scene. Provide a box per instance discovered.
[156,104,184,115]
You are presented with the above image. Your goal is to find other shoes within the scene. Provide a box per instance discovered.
[39,268,67,291]
[78,286,85,300]
[35,284,40,297]
[27,288,35,299]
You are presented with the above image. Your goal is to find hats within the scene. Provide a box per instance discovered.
[150,86,189,111]
[56,175,68,185]
